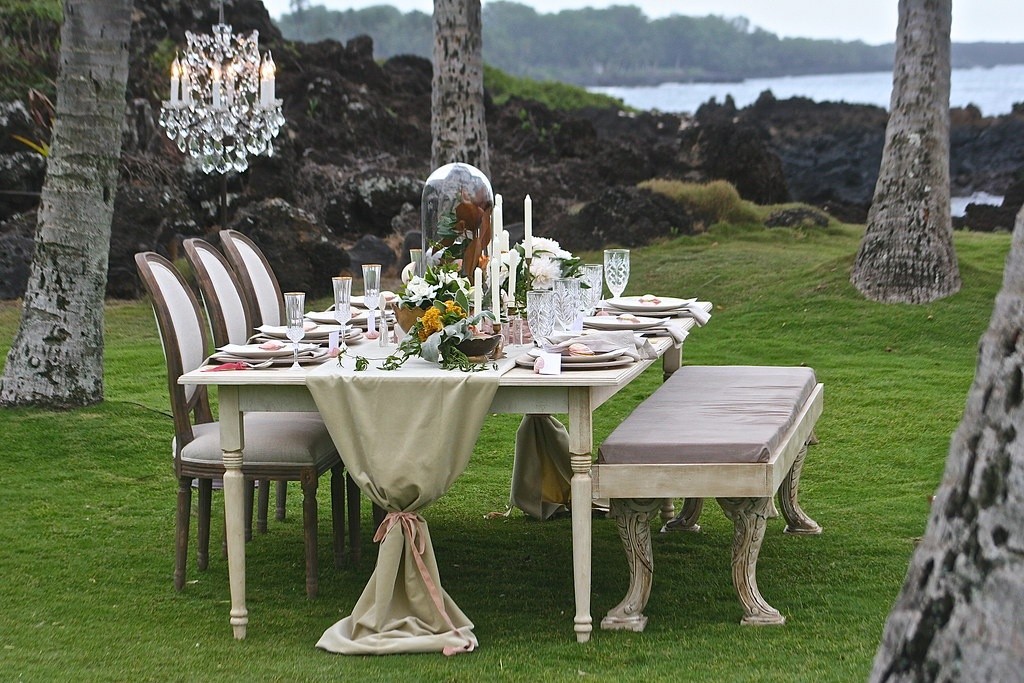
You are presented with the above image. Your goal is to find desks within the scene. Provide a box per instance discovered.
[177,301,713,642]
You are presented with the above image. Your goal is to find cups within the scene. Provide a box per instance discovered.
[410,249,422,276]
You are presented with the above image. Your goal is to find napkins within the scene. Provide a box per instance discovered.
[354,318,396,326]
[324,301,400,312]
[609,297,711,328]
[539,330,659,362]
[246,328,363,345]
[199,348,330,369]
[582,325,689,348]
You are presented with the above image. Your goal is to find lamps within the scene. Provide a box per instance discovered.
[158,0,285,176]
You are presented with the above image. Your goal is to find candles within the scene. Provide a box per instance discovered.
[474,267,483,330]
[491,193,517,323]
[525,194,532,258]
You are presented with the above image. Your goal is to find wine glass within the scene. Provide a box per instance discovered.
[603,248,631,298]
[362,265,382,314]
[578,262,603,317]
[284,292,306,371]
[332,277,352,351]
[527,290,555,348]
[553,278,580,334]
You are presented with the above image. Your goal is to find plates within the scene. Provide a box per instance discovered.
[216,343,319,358]
[607,296,690,312]
[316,324,394,329]
[215,354,330,363]
[303,311,382,323]
[583,316,670,330]
[515,356,635,367]
[582,328,667,334]
[610,311,691,316]
[256,335,364,344]
[254,324,341,338]
[527,349,628,362]
[351,294,398,307]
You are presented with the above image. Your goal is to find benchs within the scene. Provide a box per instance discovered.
[591,365,824,632]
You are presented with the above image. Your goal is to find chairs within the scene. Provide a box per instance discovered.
[182,237,381,568]
[218,229,288,530]
[134,251,346,596]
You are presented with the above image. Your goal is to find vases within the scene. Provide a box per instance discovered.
[390,301,434,334]
[457,333,502,363]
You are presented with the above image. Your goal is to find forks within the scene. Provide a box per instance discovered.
[243,357,273,369]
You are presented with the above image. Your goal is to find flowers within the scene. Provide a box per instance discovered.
[392,238,469,306]
[520,235,592,289]
[479,250,563,303]
[337,300,478,372]
[432,183,492,264]
